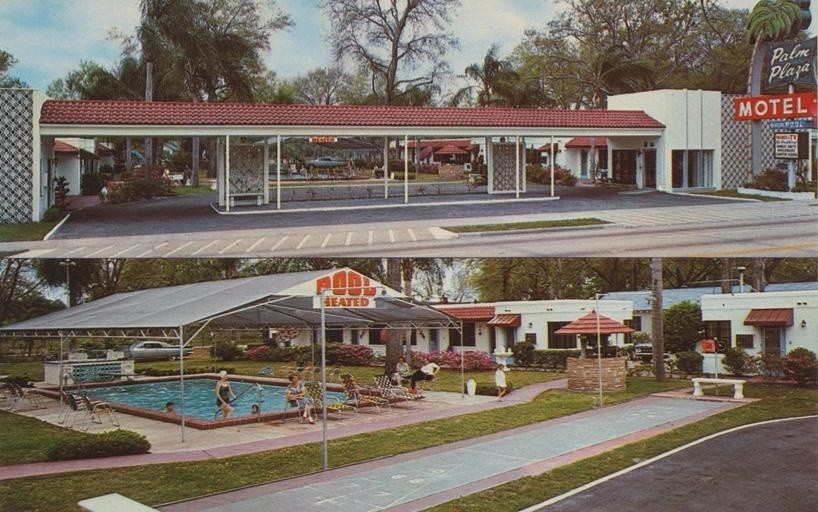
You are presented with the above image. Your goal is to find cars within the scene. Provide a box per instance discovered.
[303,154,347,169]
[125,340,195,362]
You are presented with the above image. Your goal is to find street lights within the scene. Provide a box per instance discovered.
[318,287,336,470]
[737,265,746,292]
[59,258,77,310]
[210,332,218,373]
[592,293,608,408]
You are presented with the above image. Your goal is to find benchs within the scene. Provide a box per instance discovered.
[268,178,476,204]
[691,377,746,399]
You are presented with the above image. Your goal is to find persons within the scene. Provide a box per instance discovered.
[494,361,510,401]
[376,162,384,178]
[249,403,260,413]
[394,355,410,388]
[370,163,377,177]
[285,374,316,423]
[407,357,440,390]
[300,162,312,181]
[214,370,237,420]
[163,401,176,414]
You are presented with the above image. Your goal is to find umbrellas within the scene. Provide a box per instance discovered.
[434,144,468,157]
[554,307,634,335]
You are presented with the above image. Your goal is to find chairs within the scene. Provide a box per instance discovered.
[1,371,122,432]
[280,370,427,426]
